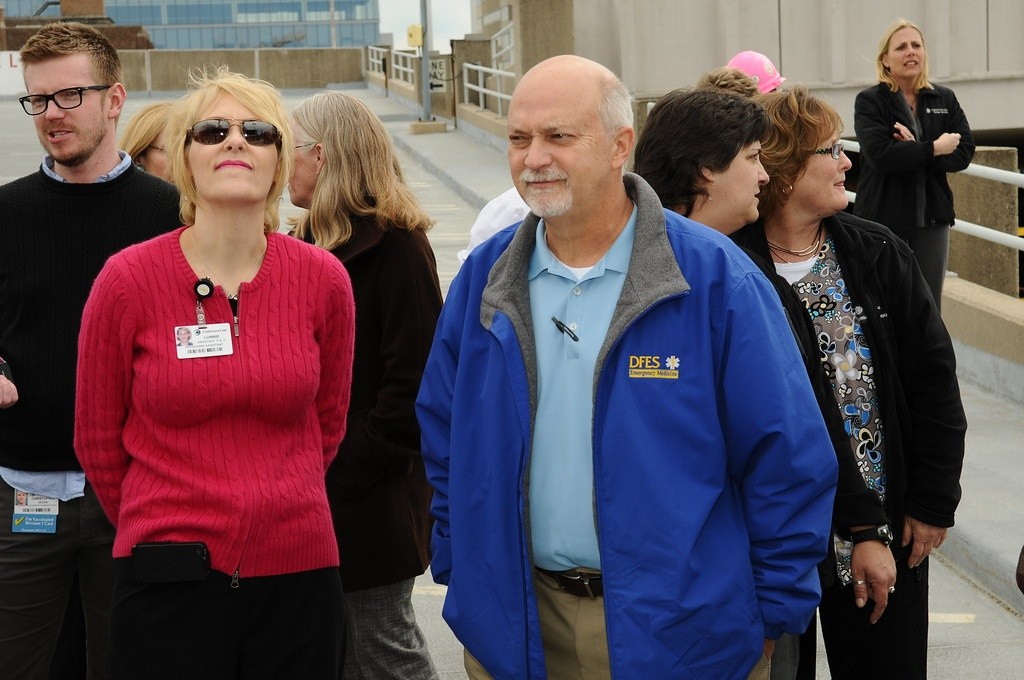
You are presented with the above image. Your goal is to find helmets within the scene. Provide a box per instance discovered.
[726,51,786,94]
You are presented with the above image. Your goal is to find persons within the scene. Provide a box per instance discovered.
[177,328,192,348]
[0,22,195,679]
[120,102,176,181]
[854,17,975,316]
[632,51,969,680]
[415,55,838,680]
[287,90,444,680]
[14,491,28,505]
[71,59,355,680]
[634,85,770,236]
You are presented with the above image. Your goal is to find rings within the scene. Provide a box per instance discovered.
[853,579,865,584]
[888,585,896,593]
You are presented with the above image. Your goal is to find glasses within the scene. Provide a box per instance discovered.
[812,142,845,160]
[184,117,282,159]
[18,85,112,116]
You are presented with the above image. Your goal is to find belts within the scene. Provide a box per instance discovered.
[534,565,603,600]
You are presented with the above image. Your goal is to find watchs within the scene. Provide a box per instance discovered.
[849,524,895,547]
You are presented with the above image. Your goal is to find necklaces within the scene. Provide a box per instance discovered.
[767,222,826,266]
[908,98,917,111]
